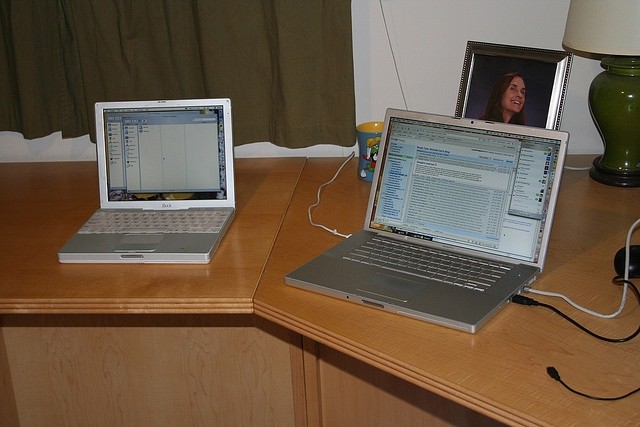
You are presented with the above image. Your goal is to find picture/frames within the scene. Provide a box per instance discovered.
[455,41,573,130]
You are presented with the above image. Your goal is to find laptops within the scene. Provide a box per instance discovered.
[285,109,570,333]
[58,98,236,263]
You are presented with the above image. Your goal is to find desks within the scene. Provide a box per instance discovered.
[0,157,308,426]
[253,154,640,426]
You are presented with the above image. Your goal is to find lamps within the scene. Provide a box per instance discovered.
[562,0,640,188]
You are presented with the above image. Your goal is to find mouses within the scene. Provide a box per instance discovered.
[614,246,640,278]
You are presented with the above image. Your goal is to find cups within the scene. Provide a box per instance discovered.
[355,122,385,182]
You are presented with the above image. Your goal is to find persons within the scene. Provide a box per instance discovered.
[479,72,527,125]
